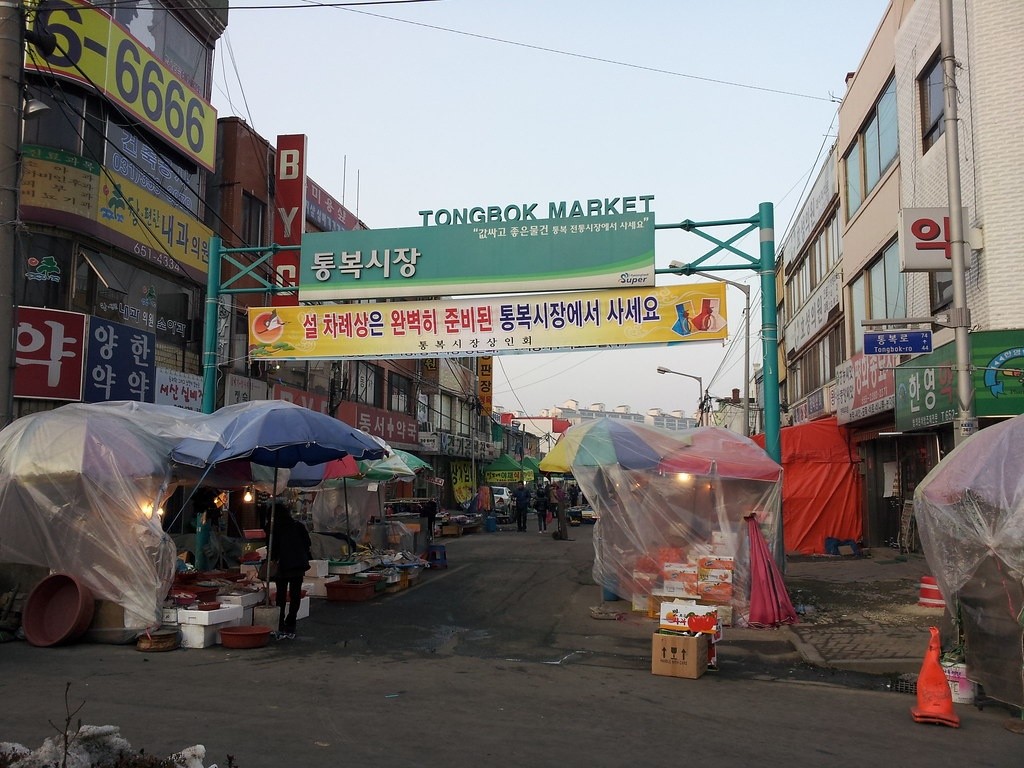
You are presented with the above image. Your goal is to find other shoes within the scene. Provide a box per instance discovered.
[544,530,547,533]
[523,527,527,532]
[278,620,286,632]
[287,620,296,633]
[517,528,522,532]
[539,531,542,534]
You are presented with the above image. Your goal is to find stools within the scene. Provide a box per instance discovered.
[429,543,447,570]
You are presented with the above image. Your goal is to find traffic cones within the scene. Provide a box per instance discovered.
[909,625,961,728]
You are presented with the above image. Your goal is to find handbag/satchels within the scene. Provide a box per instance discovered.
[545,511,552,524]
[258,559,279,580]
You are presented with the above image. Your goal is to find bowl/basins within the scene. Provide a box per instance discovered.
[218,626,273,649]
[22,573,94,648]
[198,602,221,611]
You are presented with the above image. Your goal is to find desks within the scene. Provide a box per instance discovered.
[438,523,483,536]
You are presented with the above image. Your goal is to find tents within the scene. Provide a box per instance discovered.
[749,417,863,555]
[485,454,563,485]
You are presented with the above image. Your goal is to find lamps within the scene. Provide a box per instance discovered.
[22,88,52,119]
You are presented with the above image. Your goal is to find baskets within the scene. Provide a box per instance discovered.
[136,631,180,651]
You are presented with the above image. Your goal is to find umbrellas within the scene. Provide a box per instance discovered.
[0,400,436,587]
[538,416,783,483]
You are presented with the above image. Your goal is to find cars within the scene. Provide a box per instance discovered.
[491,486,514,513]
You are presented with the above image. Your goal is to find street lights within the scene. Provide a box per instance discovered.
[657,366,703,427]
[669,260,750,438]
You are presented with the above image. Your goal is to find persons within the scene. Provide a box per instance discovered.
[593,468,649,601]
[514,480,579,533]
[265,504,314,640]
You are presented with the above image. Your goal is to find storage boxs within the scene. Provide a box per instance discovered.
[161,564,310,649]
[302,555,419,600]
[633,510,774,679]
